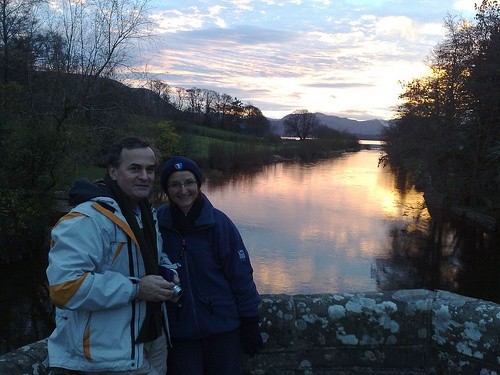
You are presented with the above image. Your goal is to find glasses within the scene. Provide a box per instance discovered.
[166,180,198,188]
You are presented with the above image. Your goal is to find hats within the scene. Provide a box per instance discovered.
[161,156,203,192]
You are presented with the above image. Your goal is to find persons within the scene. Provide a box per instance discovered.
[155,154,266,375]
[44,136,182,375]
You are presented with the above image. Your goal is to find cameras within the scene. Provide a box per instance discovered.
[168,284,183,298]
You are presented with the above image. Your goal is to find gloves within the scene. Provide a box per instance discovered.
[239,318,264,357]
